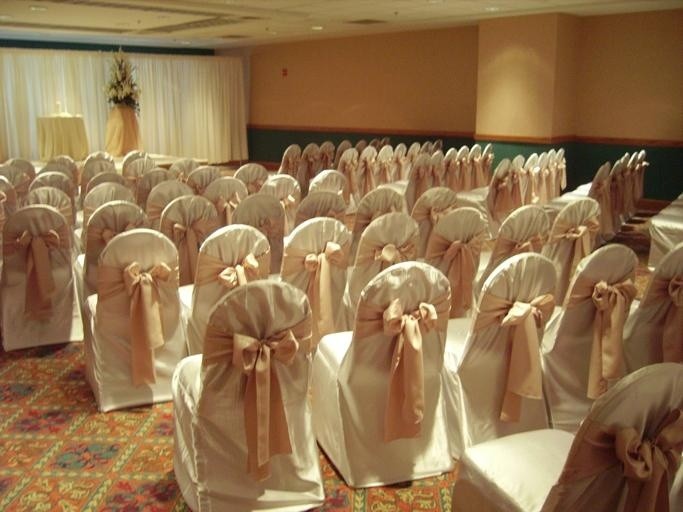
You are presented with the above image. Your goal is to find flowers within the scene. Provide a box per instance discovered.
[104,39,141,118]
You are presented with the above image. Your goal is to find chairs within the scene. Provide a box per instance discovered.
[0,137,682,511]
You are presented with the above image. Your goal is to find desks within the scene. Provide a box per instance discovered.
[34,113,89,163]
[103,105,143,159]
